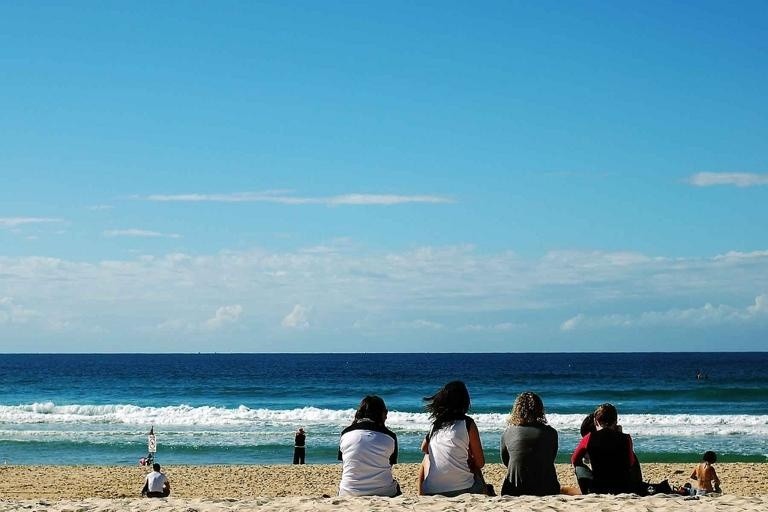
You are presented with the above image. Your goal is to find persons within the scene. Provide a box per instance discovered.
[580,413,642,490]
[690,451,720,492]
[417,381,497,497]
[572,403,633,495]
[499,391,561,494]
[293,427,305,464]
[338,396,394,495]
[142,463,170,497]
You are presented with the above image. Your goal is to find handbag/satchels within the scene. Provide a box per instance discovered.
[642,480,672,495]
